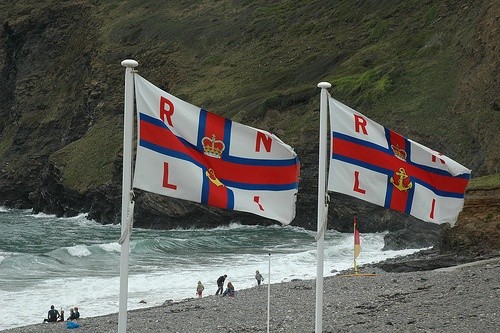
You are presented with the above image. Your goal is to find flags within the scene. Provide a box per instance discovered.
[133,74,299,225]
[327,94,471,228]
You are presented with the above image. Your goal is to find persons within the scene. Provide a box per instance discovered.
[58,307,64,322]
[197,281,204,298]
[255,270,264,287]
[67,307,80,321]
[43,305,60,323]
[215,275,227,295]
[222,282,234,296]
